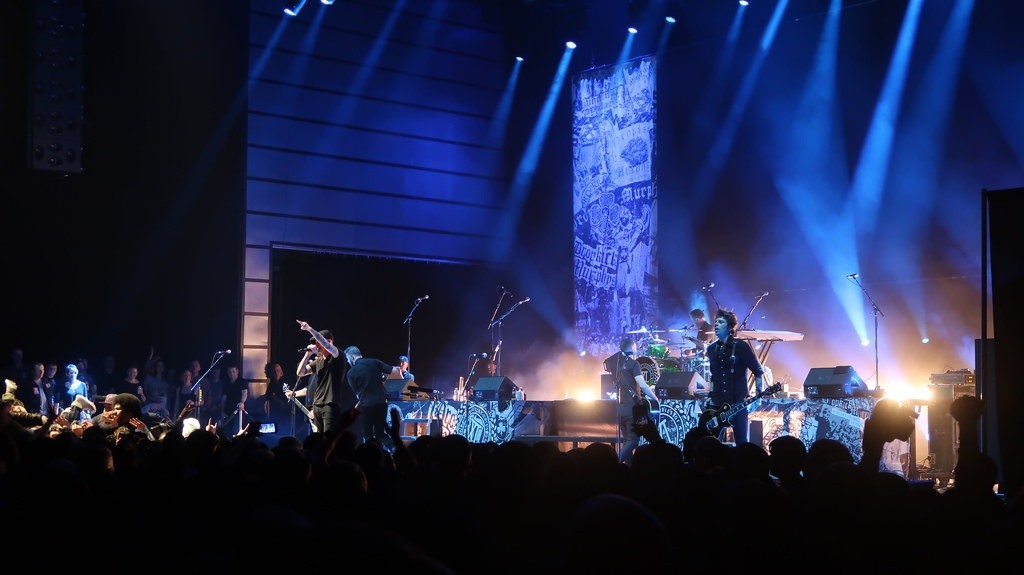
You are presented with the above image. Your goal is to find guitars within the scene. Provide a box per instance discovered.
[641,396,660,429]
[698,382,783,439]
[283,383,318,433]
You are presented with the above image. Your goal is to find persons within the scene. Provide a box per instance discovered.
[344,346,398,443]
[619,339,661,461]
[0,349,1024,575]
[707,309,764,446]
[296,320,345,431]
[284,351,323,436]
[684,309,716,355]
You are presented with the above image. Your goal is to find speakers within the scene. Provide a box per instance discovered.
[383,378,429,401]
[473,375,526,401]
[601,374,618,401]
[803,368,868,398]
[655,372,709,399]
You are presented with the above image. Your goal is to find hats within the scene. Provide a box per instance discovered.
[310,330,335,341]
[98,393,119,405]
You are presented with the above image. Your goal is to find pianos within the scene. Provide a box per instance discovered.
[716,329,805,392]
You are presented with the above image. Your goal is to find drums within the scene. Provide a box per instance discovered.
[680,357,710,382]
[636,356,680,387]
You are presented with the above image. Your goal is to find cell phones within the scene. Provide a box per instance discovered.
[258,422,278,433]
[633,404,648,424]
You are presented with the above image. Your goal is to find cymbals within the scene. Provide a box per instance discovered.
[655,342,688,346]
[706,332,716,335]
[650,338,667,343]
[626,329,666,333]
[669,328,703,332]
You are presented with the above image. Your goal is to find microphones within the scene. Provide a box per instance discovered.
[470,353,487,358]
[754,291,769,298]
[298,348,318,353]
[517,296,530,304]
[499,285,513,298]
[218,349,232,354]
[847,273,859,278]
[702,283,715,289]
[417,294,429,300]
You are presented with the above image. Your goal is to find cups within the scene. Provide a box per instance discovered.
[517,392,524,400]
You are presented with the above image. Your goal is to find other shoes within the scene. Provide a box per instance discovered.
[5,379,17,394]
[71,394,96,414]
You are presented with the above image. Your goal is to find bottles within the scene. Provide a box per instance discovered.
[512,386,517,401]
[53,401,59,416]
[518,386,523,391]
[138,386,142,394]
[454,387,459,401]
